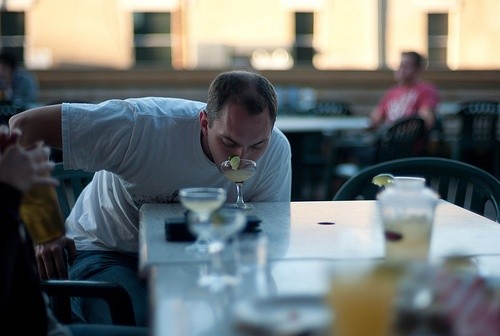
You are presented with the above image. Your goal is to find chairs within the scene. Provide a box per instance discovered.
[374,100,500,166]
[332,158,500,223]
[0,148,150,336]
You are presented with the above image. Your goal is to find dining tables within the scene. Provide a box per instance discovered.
[139,202,500,336]
[275,115,370,201]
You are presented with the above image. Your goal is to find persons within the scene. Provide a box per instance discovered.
[327,52,439,164]
[0,125,61,193]
[9,71,292,328]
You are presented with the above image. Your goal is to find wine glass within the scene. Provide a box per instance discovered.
[220,159,257,209]
[178,185,228,251]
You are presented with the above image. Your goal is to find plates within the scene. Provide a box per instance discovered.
[227,292,337,336]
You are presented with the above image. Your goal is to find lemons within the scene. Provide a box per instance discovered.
[230,155,240,170]
[372,174,395,187]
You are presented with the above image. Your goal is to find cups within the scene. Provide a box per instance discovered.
[376,175,439,259]
[18,139,65,245]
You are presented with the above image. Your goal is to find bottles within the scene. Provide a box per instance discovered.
[323,260,406,335]
